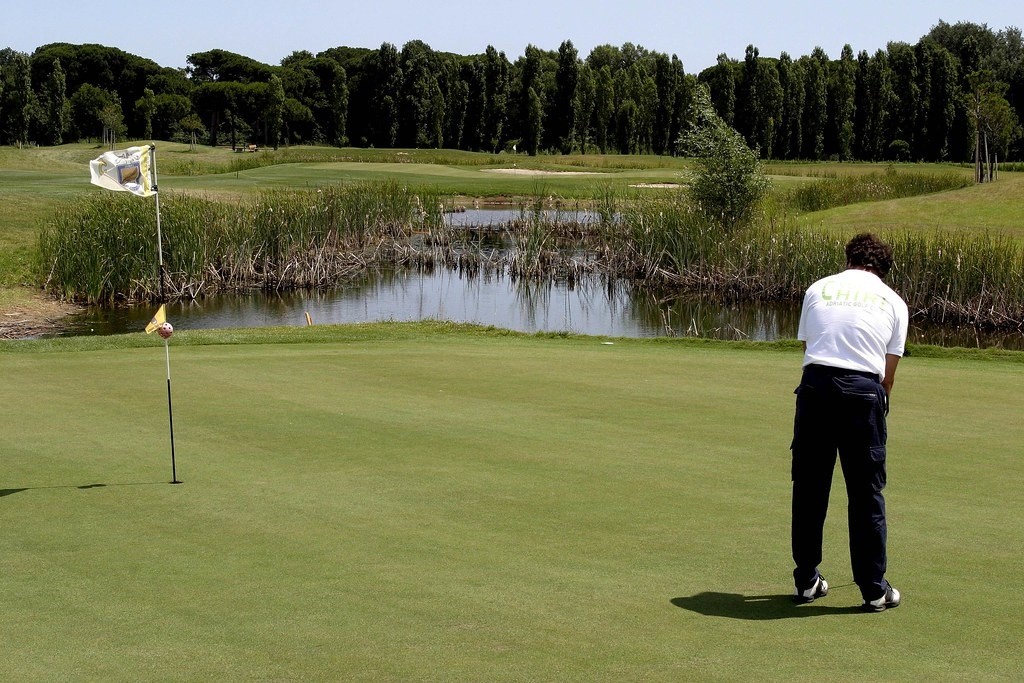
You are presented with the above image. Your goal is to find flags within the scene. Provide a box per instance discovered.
[89,145,157,198]
[145,303,166,335]
[513,145,516,150]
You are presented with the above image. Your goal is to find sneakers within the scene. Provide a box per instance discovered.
[862,580,901,612]
[797,574,828,603]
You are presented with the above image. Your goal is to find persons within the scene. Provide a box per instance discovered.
[789,232,908,613]
